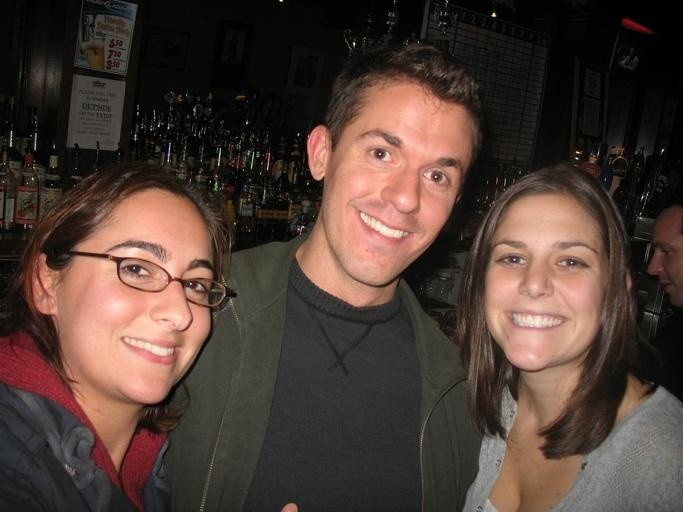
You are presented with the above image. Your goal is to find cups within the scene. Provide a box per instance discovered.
[423,272,452,302]
[79,39,106,71]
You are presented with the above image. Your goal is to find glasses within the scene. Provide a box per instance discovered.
[57,249,238,310]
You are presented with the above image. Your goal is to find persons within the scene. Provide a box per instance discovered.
[0,158,238,511]
[456,162,682,512]
[143,36,484,511]
[643,197,682,310]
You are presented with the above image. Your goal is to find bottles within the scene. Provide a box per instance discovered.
[477,171,522,210]
[0,107,104,231]
[127,91,321,245]
[580,155,601,178]
[632,146,645,173]
